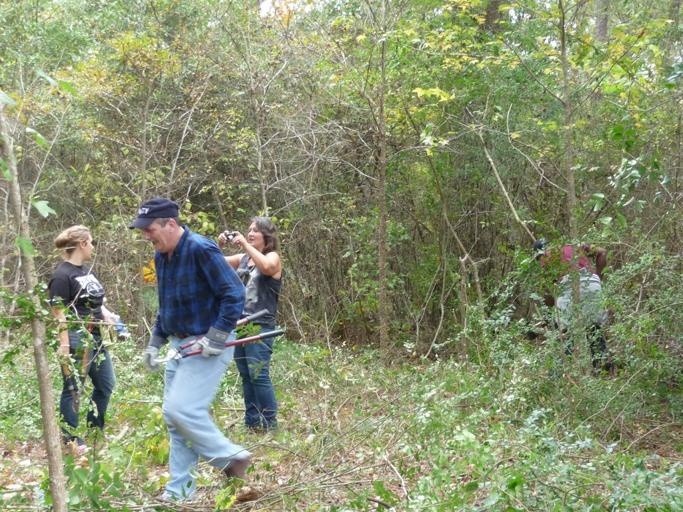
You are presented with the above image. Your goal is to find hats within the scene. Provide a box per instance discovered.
[127,197,179,229]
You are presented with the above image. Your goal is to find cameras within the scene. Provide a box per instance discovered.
[224,231,236,241]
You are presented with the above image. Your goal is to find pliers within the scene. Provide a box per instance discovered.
[43,300,95,413]
[152,308,283,363]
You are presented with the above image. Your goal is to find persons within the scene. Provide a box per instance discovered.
[48,224,129,454]
[216,216,282,432]
[527,236,611,378]
[129,195,252,504]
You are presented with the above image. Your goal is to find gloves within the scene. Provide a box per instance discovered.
[143,335,169,373]
[190,326,231,358]
